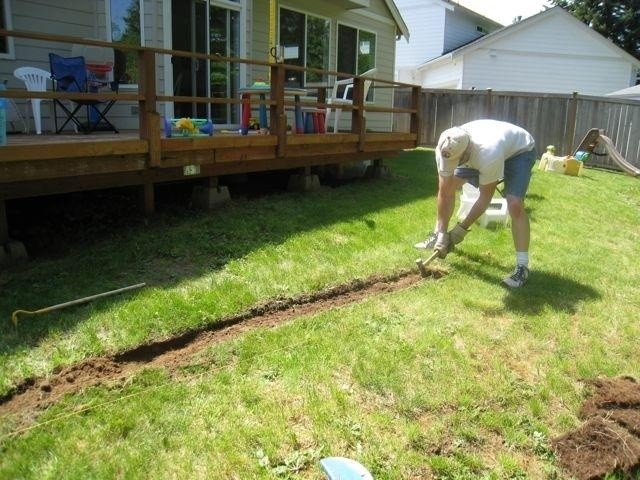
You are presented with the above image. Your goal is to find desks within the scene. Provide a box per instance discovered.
[236,86,307,135]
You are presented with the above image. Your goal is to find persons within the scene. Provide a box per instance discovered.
[415,118,536,288]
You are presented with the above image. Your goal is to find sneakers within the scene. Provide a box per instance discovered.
[500,264,530,291]
[413,234,437,251]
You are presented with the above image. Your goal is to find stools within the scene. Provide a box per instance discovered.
[302,109,326,134]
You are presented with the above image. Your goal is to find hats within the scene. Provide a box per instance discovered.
[436,126,470,178]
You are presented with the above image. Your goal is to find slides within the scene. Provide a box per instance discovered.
[595,135,640,177]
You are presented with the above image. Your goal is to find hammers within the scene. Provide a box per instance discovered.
[416,249,442,277]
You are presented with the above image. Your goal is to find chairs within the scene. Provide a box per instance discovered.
[13,52,120,135]
[323,68,377,133]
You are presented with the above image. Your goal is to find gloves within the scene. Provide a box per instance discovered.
[450,223,471,245]
[435,232,451,259]
